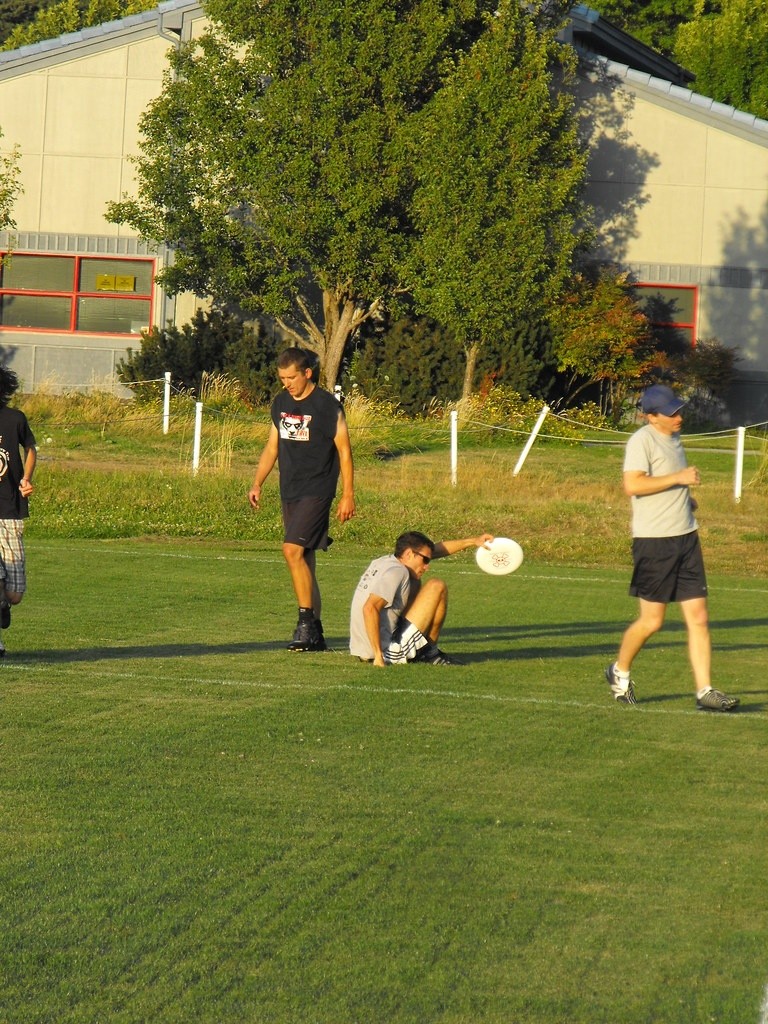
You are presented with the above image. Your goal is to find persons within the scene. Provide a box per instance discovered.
[350,531,495,666]
[605,383,742,710]
[0,366,37,652]
[249,348,357,652]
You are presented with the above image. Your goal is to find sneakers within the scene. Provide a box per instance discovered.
[286,607,317,652]
[604,661,637,705]
[306,621,328,652]
[426,649,463,667]
[696,689,740,712]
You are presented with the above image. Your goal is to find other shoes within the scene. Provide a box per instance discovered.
[0,643,5,657]
[0,599,10,630]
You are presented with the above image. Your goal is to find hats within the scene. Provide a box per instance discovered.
[642,385,685,417]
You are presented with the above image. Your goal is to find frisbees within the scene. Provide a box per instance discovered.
[475,538,524,575]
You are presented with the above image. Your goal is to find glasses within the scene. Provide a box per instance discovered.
[412,549,431,564]
